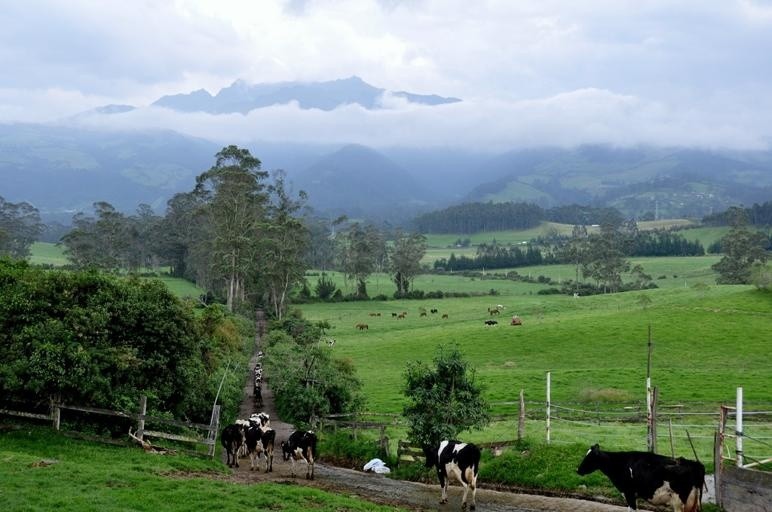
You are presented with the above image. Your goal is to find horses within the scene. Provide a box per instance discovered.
[355,324,369,331]
[490,310,501,316]
[392,308,449,320]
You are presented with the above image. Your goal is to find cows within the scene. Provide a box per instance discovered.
[484,320,498,327]
[575,443,706,512]
[420,439,481,512]
[220,412,276,474]
[280,429,318,481]
